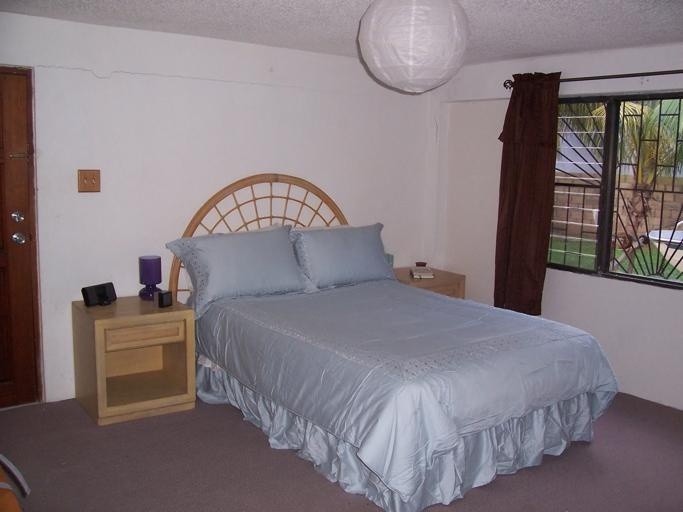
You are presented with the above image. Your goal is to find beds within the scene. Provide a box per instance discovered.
[169,174,618,512]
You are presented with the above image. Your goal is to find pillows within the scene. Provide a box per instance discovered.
[287,223,395,289]
[165,224,318,319]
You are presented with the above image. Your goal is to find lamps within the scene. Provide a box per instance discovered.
[359,0,471,93]
[138,255,162,300]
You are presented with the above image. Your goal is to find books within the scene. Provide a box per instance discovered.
[409,266,434,279]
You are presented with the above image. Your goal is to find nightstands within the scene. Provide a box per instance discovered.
[392,266,466,299]
[72,294,197,426]
